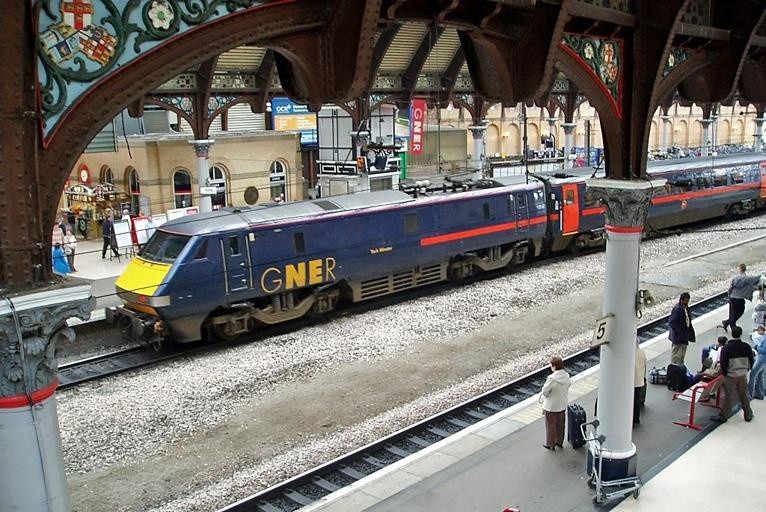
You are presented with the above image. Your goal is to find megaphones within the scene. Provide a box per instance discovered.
[643,290,654,308]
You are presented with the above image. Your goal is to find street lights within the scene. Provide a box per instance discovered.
[518,106,530,187]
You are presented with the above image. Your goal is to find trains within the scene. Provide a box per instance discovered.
[104,151,766,352]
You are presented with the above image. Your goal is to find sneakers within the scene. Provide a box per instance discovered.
[753,395,764,400]
[722,319,729,333]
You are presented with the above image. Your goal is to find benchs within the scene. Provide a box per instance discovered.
[671,371,725,431]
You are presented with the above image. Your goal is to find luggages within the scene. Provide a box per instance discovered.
[701,343,717,367]
[647,366,668,385]
[566,402,588,449]
[667,362,687,392]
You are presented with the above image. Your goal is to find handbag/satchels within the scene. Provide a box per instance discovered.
[686,368,696,388]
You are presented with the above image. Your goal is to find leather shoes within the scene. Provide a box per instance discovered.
[745,413,754,422]
[557,443,563,449]
[541,443,557,451]
[710,413,727,423]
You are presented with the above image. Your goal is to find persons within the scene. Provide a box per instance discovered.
[667,293,696,366]
[542,357,571,450]
[50,208,156,276]
[633,338,647,426]
[698,264,766,423]
[576,155,585,166]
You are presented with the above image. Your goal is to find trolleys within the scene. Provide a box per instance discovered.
[580,417,643,506]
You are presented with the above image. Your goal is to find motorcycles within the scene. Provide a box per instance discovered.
[649,144,752,160]
